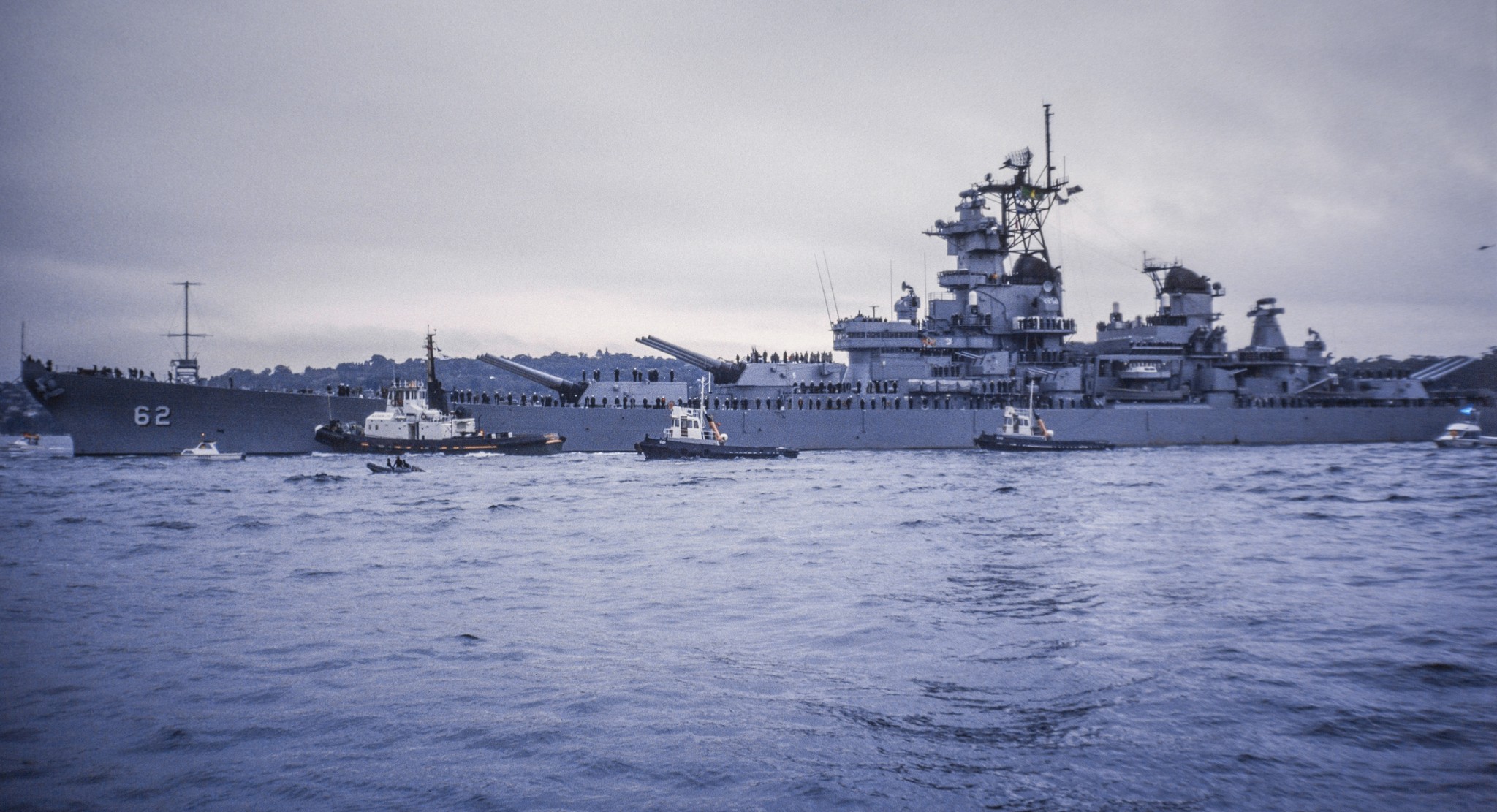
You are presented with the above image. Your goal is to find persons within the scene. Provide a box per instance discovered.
[736,349,833,367]
[1020,317,1075,363]
[1231,350,1440,408]
[792,377,897,394]
[837,313,927,329]
[387,456,409,469]
[228,376,234,389]
[450,388,1091,409]
[326,383,389,398]
[581,366,675,382]
[901,347,962,377]
[27,353,174,383]
[951,311,991,326]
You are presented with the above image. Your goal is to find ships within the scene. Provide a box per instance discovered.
[19,98,1497,459]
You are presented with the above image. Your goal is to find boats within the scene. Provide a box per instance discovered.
[967,380,1116,452]
[314,329,569,458]
[1435,407,1482,448]
[633,371,803,461]
[365,458,436,477]
[14,435,41,448]
